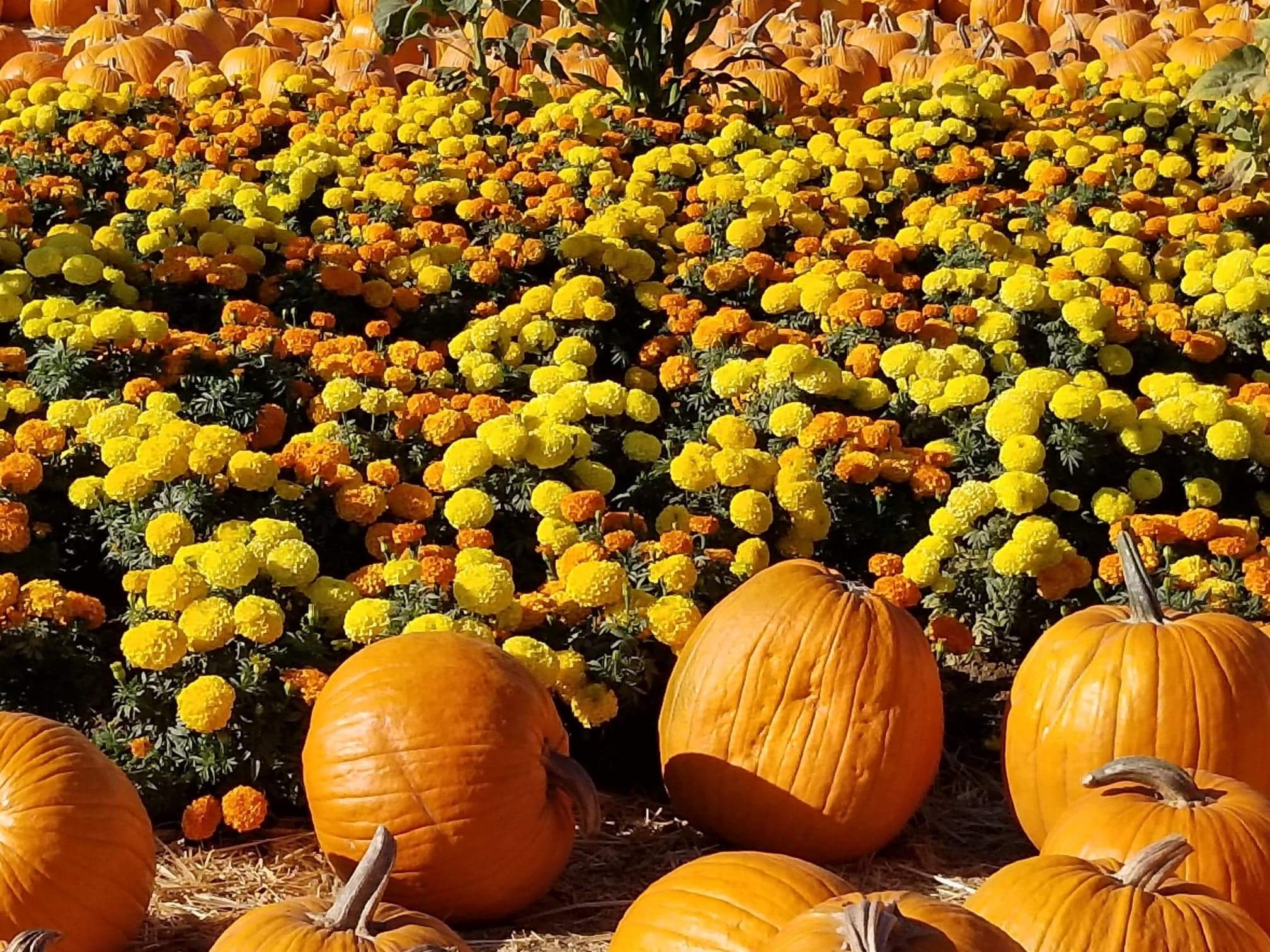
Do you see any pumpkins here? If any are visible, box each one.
[0,0,1270,123]
[0,714,155,952]
[207,531,1270,952]
